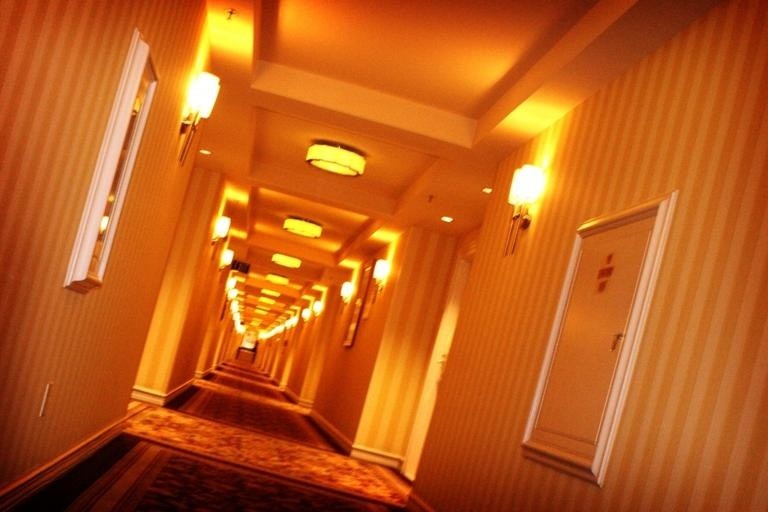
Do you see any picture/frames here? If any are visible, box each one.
[63,27,160,294]
[520,187,681,488]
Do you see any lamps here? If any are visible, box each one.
[176,72,546,345]
